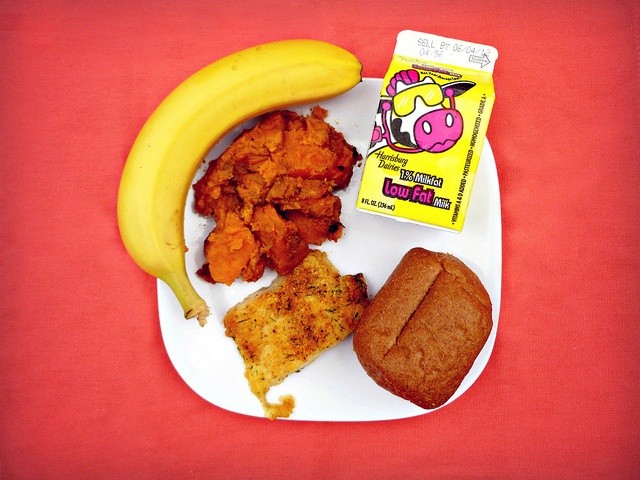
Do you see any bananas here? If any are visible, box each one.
[116,38,363,318]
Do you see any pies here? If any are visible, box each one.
[224,254,367,415]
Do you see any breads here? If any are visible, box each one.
[356,249,492,403]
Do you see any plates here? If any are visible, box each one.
[156,77,503,423]
